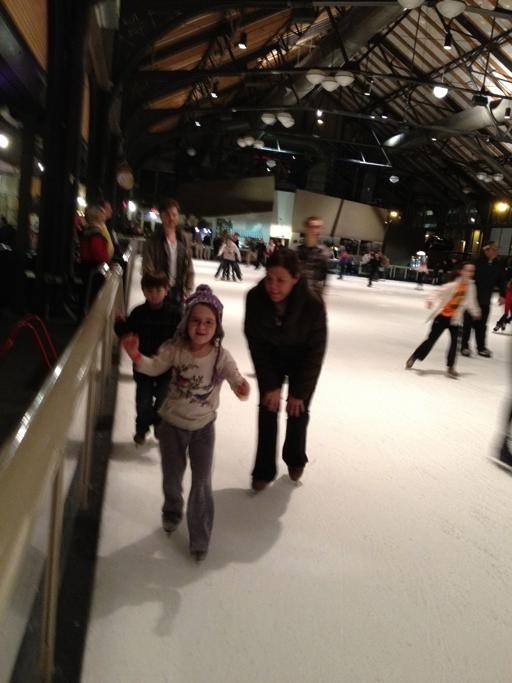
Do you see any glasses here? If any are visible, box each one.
[190,319,214,327]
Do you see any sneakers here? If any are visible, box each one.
[478,350,490,355]
[406,355,417,369]
[189,542,209,562]
[448,367,458,377]
[288,466,304,481]
[502,322,506,331]
[162,511,183,533]
[460,347,472,355]
[493,326,500,331]
[133,427,150,443]
[252,479,269,491]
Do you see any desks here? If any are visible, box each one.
[377,262,441,287]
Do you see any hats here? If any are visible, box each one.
[174,284,226,388]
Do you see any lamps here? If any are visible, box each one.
[112,129,136,194]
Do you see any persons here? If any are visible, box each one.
[70,197,283,449]
[122,284,251,561]
[243,248,327,492]
[404,241,512,472]
[291,216,391,294]
